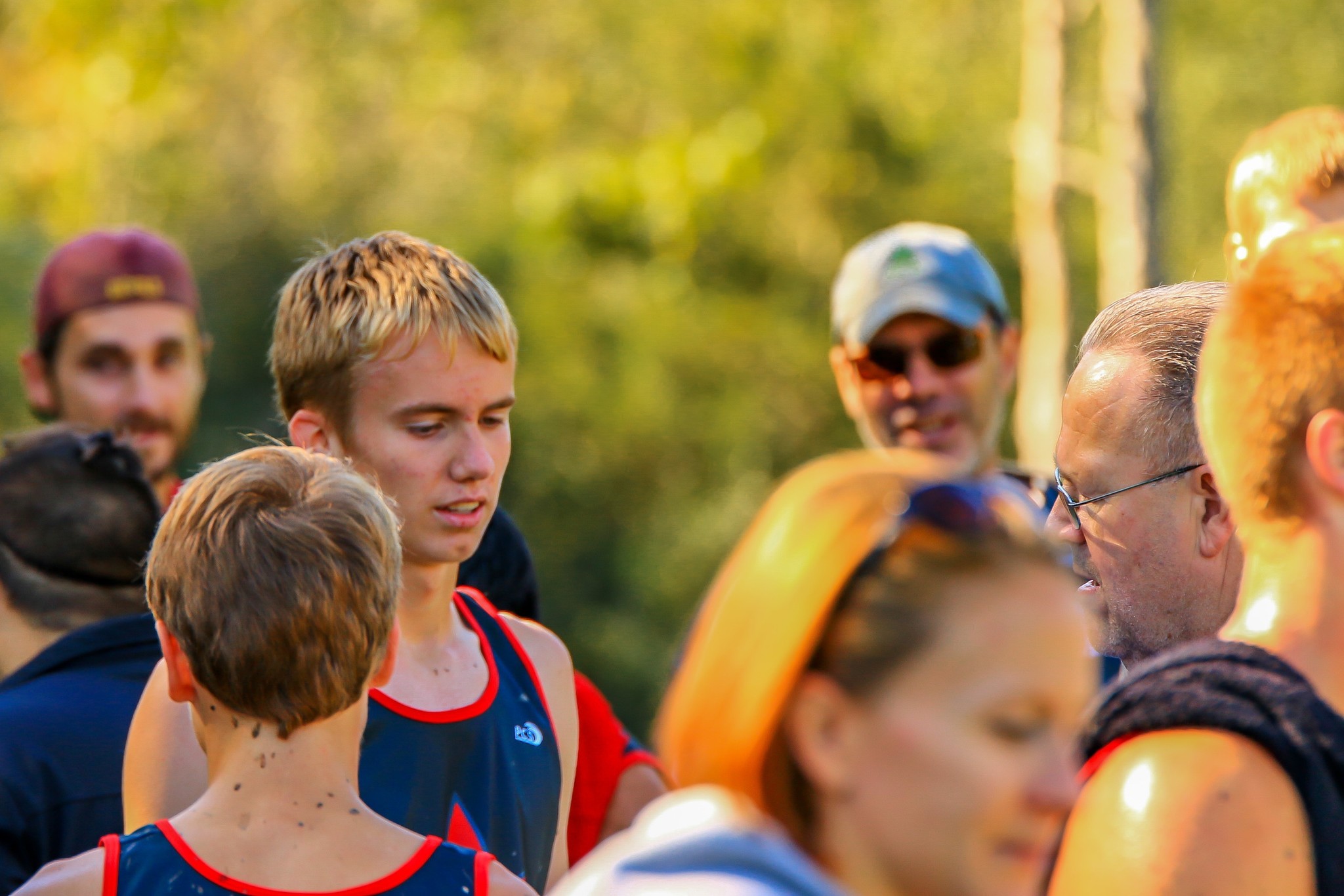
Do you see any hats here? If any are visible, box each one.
[833,220,1007,361]
[35,227,197,343]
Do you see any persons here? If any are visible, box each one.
[1,106,1344,896]
[536,446,1096,896]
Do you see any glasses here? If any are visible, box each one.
[1056,458,1204,530]
[809,475,1089,667]
[865,328,977,374]
